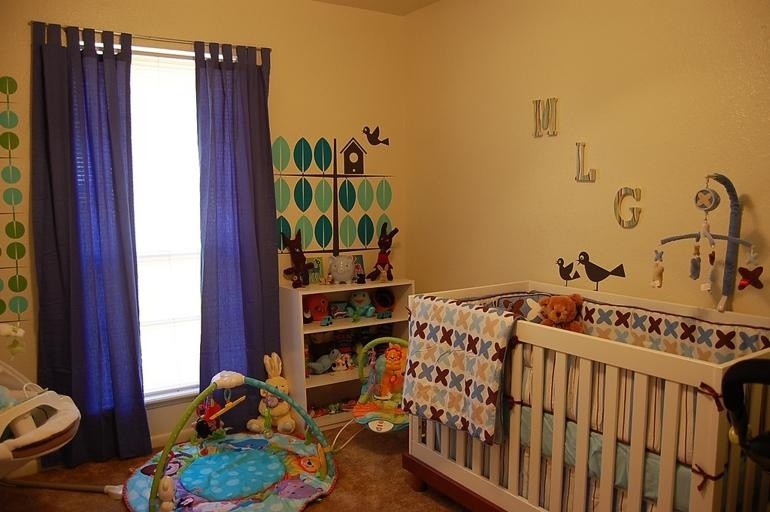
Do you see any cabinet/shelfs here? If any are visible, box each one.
[278,278,416,433]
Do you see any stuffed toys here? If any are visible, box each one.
[280,221,418,454]
[539,293,587,334]
[246,352,296,434]
[157,473,177,511]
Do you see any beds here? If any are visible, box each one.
[399,279,768,512]
